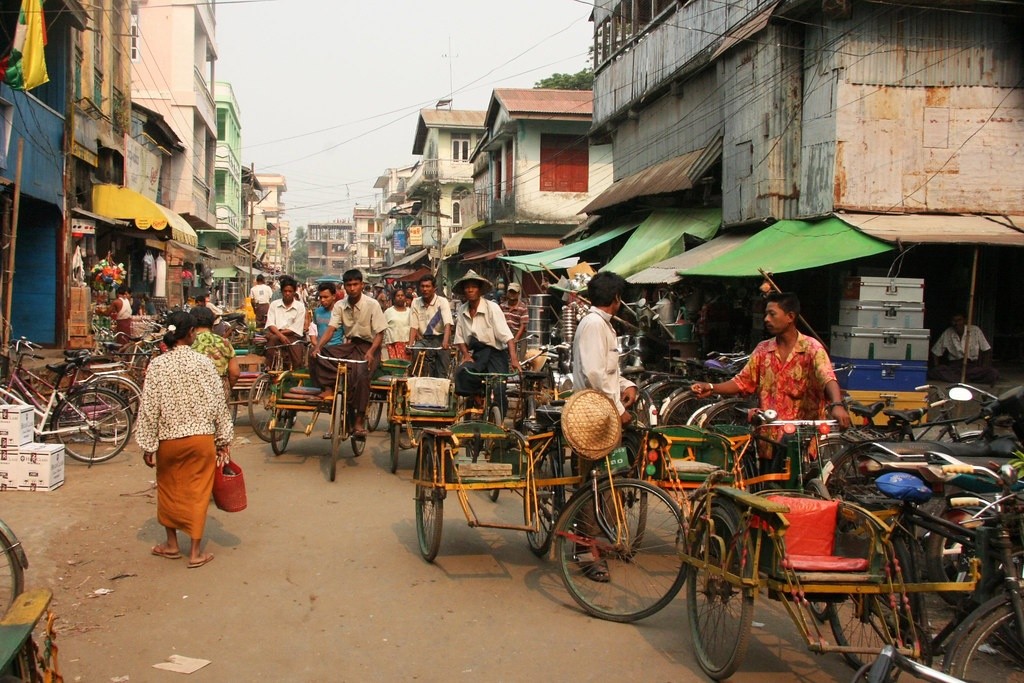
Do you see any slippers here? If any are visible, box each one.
[152,544,181,558]
[187,553,214,567]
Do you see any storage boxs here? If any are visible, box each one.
[67,285,95,348]
[0,404,65,493]
[829,276,931,427]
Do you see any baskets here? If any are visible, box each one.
[0,353,10,378]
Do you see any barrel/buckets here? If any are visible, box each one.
[528,294,551,345]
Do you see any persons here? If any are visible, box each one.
[569,272,638,581]
[306,269,388,438]
[133,311,234,569]
[404,275,454,378]
[250,274,420,335]
[313,282,351,346]
[383,288,422,361]
[195,295,224,338]
[925,312,1000,386]
[263,280,306,372]
[189,306,239,410]
[498,282,529,362]
[452,269,522,425]
[690,293,853,579]
[98,288,141,353]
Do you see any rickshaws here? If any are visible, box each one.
[227,333,1024,683]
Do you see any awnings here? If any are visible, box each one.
[837,212,1024,246]
[235,265,269,277]
[378,250,431,284]
[600,208,894,283]
[439,215,649,274]
[575,130,721,218]
[91,182,198,246]
[169,241,221,261]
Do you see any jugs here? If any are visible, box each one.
[626,297,692,343]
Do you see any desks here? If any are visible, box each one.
[669,340,699,360]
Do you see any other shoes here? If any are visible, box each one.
[354,428,367,437]
[578,552,610,582]
[594,542,618,559]
[312,391,334,400]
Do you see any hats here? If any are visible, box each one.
[524,349,549,371]
[508,282,520,292]
[562,388,621,461]
[451,270,493,295]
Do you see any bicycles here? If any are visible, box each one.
[0,315,166,468]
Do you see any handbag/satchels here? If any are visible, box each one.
[212,450,247,512]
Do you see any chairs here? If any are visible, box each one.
[753,492,892,585]
[273,373,329,412]
[434,421,523,484]
[400,376,455,417]
[654,423,733,482]
[371,358,409,389]
[236,354,266,390]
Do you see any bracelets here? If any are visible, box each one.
[708,383,714,396]
